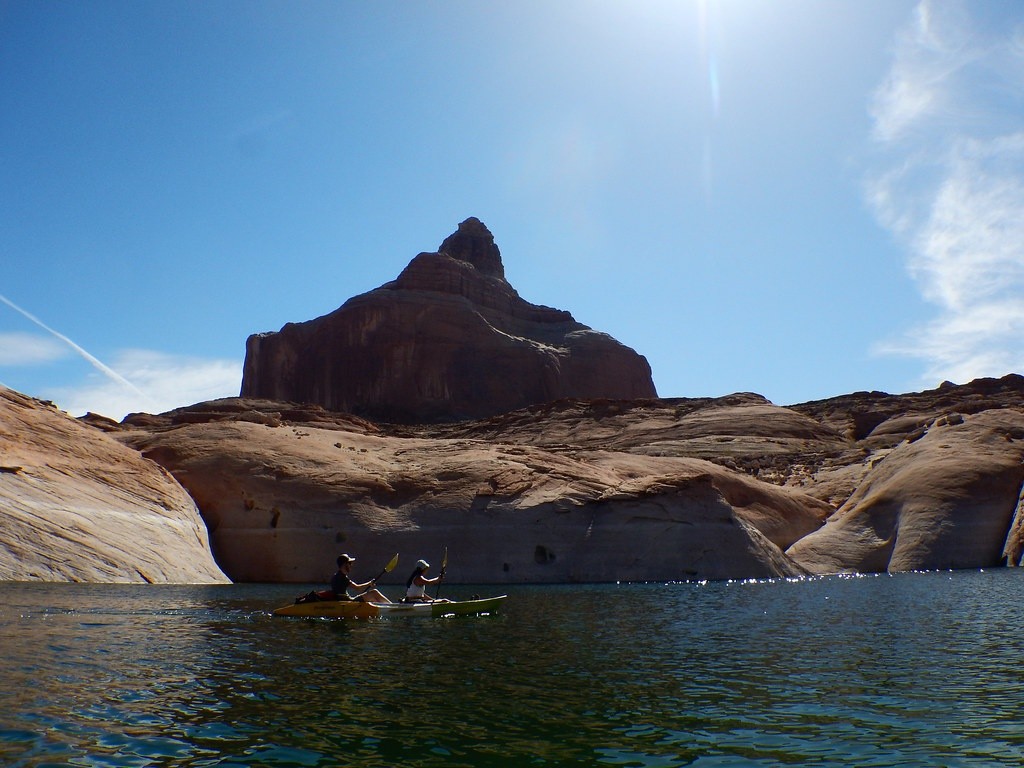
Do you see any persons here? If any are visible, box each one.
[330,554,405,603]
[405,560,480,603]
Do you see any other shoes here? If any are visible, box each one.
[475,594,480,600]
[470,595,475,600]
[398,598,402,603]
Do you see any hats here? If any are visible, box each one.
[337,554,356,565]
[416,560,430,570]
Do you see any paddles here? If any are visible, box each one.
[436,546,448,600]
[362,553,398,593]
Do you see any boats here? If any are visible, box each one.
[271,594,509,621]
